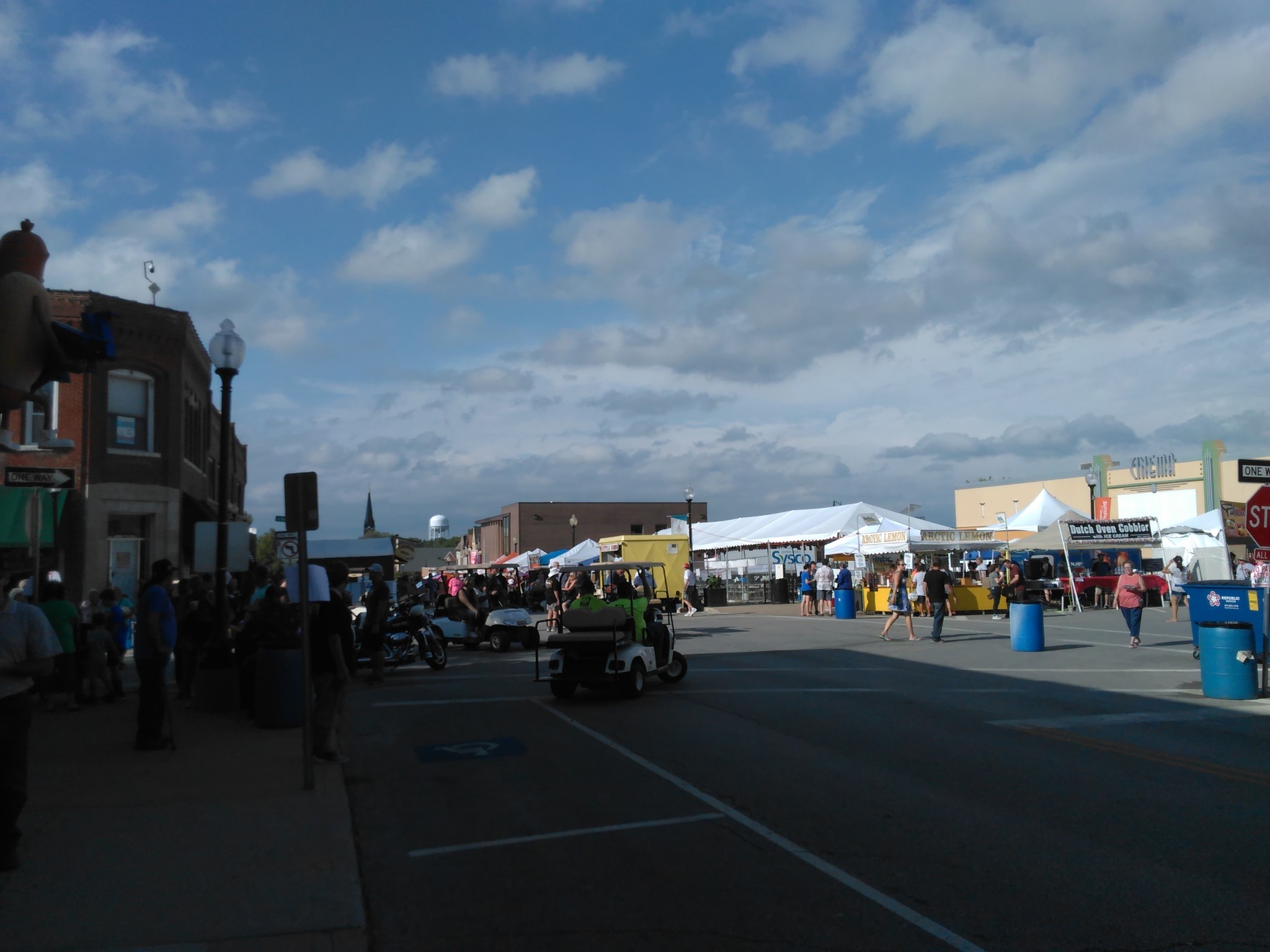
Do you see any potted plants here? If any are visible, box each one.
[702,573,731,607]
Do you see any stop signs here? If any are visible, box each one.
[1244,484,1270,548]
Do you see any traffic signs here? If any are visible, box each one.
[1237,458,1270,485]
[4,467,75,490]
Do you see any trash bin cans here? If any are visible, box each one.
[1198,620,1259,700]
[1009,599,1045,652]
[834,587,856,619]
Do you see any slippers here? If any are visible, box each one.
[1129,642,1143,648]
[1166,619,1178,623]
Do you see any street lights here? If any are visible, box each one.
[206,318,245,664]
[1086,465,1099,520]
[512,537,518,554]
[568,513,578,548]
[684,482,695,575]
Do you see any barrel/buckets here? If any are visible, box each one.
[1008,600,1045,652]
[834,587,856,619]
[1197,621,1259,700]
[771,578,789,604]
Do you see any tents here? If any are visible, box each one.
[997,508,1162,607]
[976,481,1090,532]
[652,501,957,603]
[494,538,602,575]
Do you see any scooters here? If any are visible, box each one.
[531,559,687,701]
[426,588,540,654]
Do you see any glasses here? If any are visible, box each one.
[899,564,906,566]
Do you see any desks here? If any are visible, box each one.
[1058,575,1169,609]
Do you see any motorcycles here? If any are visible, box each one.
[345,604,447,671]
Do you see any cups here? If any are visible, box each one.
[999,570,1004,577]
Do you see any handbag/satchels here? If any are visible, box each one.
[886,589,898,605]
[1185,567,1193,580]
[987,594,993,600]
[1138,598,1144,609]
[908,589,918,601]
[1244,576,1250,581]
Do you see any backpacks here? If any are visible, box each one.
[1002,585,1015,599]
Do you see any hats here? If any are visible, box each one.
[810,561,817,564]
[366,564,384,575]
[1097,553,1105,557]
[682,563,690,568]
[1235,556,1248,562]
[548,568,560,578]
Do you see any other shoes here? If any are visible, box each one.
[1094,604,1109,609]
[684,608,697,616]
[546,627,557,632]
[879,634,945,643]
[0,853,18,874]
[801,611,833,616]
[312,749,338,762]
[470,630,482,637]
[364,676,383,686]
[135,736,171,751]
[919,615,953,617]
[992,611,1009,620]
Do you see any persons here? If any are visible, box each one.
[0,561,853,689]
[1235,555,1255,580]
[132,558,183,750]
[878,558,922,641]
[1162,555,1191,622]
[1112,561,1147,648]
[309,561,349,763]
[1092,553,1111,609]
[923,557,957,643]
[883,552,1075,619]
[1230,550,1238,578]
[614,552,622,562]
[0,557,63,874]
